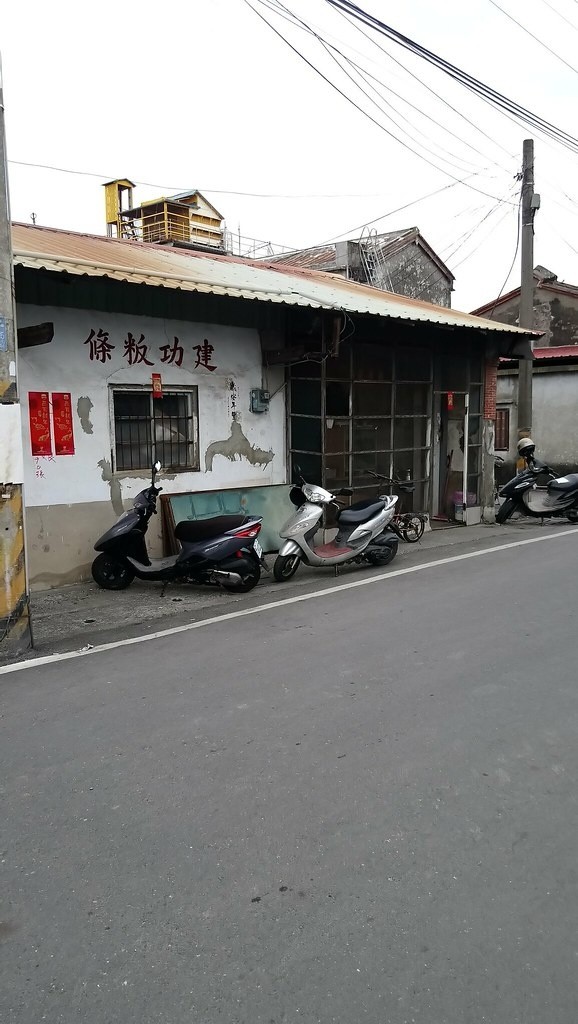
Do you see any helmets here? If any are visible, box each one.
[517,438,536,456]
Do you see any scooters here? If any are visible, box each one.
[495,452,578,524]
[273,464,400,582]
[91,459,270,598]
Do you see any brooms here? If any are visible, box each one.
[430,449,454,521]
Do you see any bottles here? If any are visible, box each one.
[405,469,410,481]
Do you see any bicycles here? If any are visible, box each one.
[367,469,427,543]
